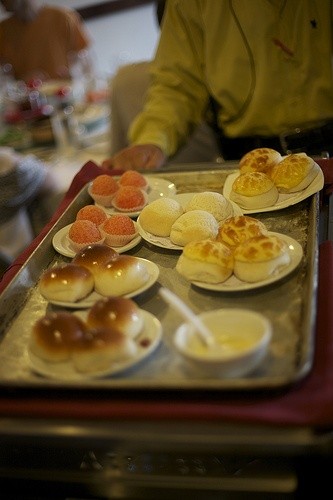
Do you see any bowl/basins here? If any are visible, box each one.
[174,309,270,378]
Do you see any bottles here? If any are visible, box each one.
[52,88,80,157]
[24,79,57,157]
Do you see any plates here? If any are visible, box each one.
[191,231,302,291]
[52,217,142,256]
[95,177,176,217]
[223,173,324,214]
[49,256,159,307]
[137,193,243,250]
[24,308,163,380]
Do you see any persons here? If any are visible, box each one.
[102,0,333,172]
[0,0,96,83]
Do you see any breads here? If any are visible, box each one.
[74,329,138,371]
[140,198,184,237]
[230,172,279,209]
[93,255,150,297]
[71,244,118,274]
[30,312,91,362]
[170,210,219,246]
[272,153,320,193]
[86,296,145,339]
[185,192,234,225]
[176,239,234,283]
[39,263,94,302]
[234,234,291,282]
[239,148,282,175]
[216,216,267,250]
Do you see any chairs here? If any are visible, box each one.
[109,60,221,167]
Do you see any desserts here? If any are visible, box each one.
[88,170,149,211]
[67,205,139,249]
